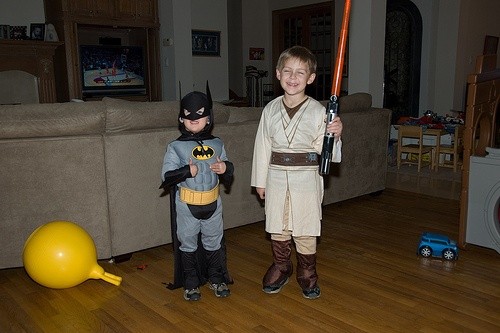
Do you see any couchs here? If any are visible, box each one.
[0,93,392,270]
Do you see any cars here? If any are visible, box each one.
[417,234,459,261]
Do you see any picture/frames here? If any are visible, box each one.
[190,28,222,57]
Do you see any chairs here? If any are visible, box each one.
[0,70,39,105]
[396,125,432,171]
[433,126,465,173]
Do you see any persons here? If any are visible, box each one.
[250,46,342,299]
[32,25,42,40]
[159,91,234,302]
[192,36,217,52]
[82,48,144,78]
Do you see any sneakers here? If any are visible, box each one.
[209,282,231,298]
[184,286,202,301]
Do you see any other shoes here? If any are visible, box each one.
[303,285,321,298]
[262,277,289,294]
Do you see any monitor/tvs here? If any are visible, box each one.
[79,44,148,94]
